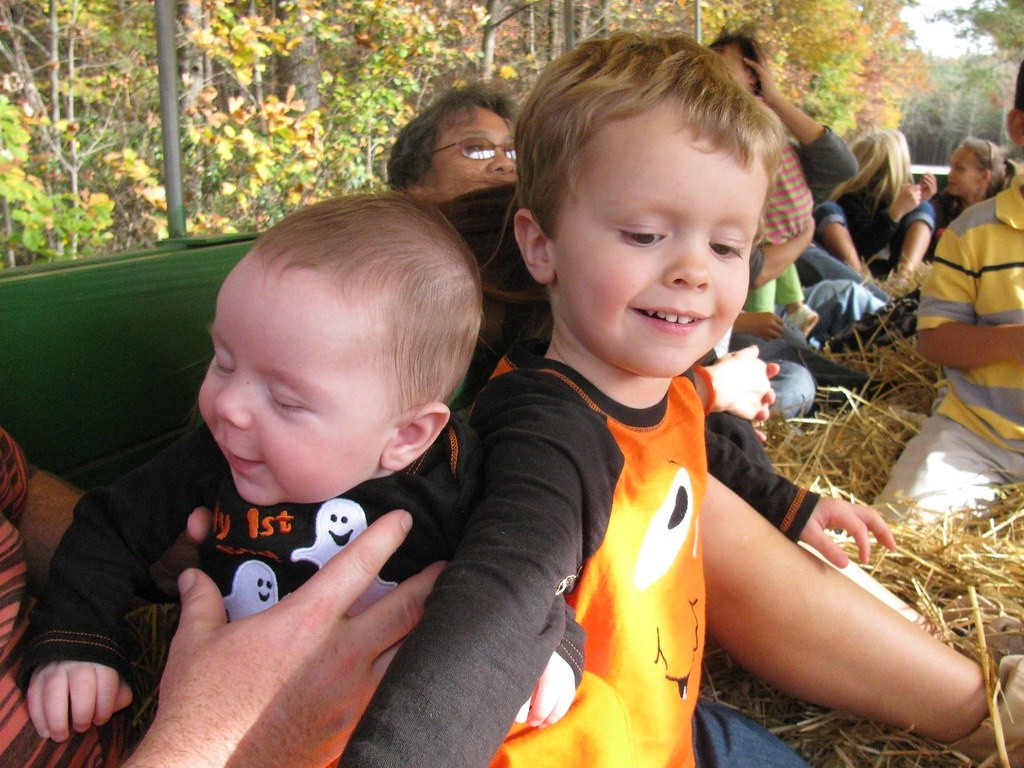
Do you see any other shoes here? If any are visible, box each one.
[783,305,820,338]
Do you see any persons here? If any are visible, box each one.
[10,191,587,742]
[0,428,448,768]
[340,30,897,768]
[708,31,913,425]
[438,184,1024,768]
[389,82,779,469]
[818,125,937,292]
[920,137,1018,262]
[875,62,1024,524]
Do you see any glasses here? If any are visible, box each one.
[432,136,516,161]
